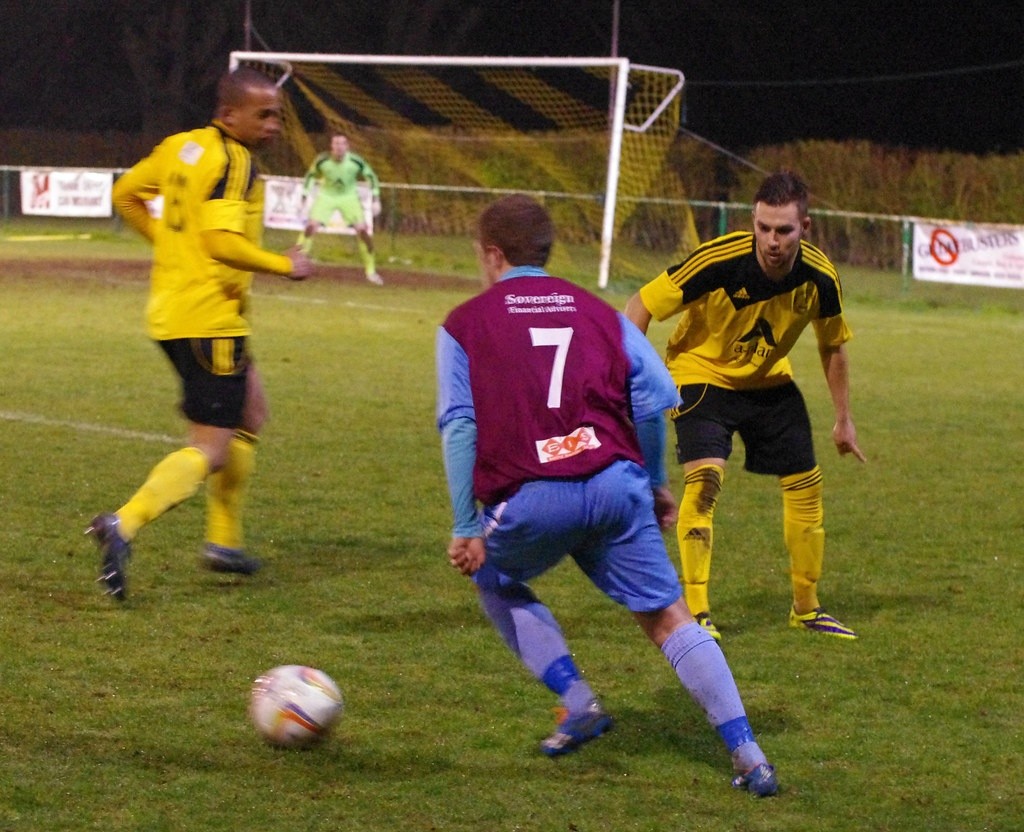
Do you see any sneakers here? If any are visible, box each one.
[542,704,612,756]
[789,604,858,639]
[205,545,258,573]
[697,614,721,640]
[86,512,130,597]
[733,762,780,796]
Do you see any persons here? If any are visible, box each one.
[296,133,385,285]
[436,193,781,800]
[84,68,319,605]
[623,170,867,641]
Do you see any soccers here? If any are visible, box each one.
[247,663,343,753]
